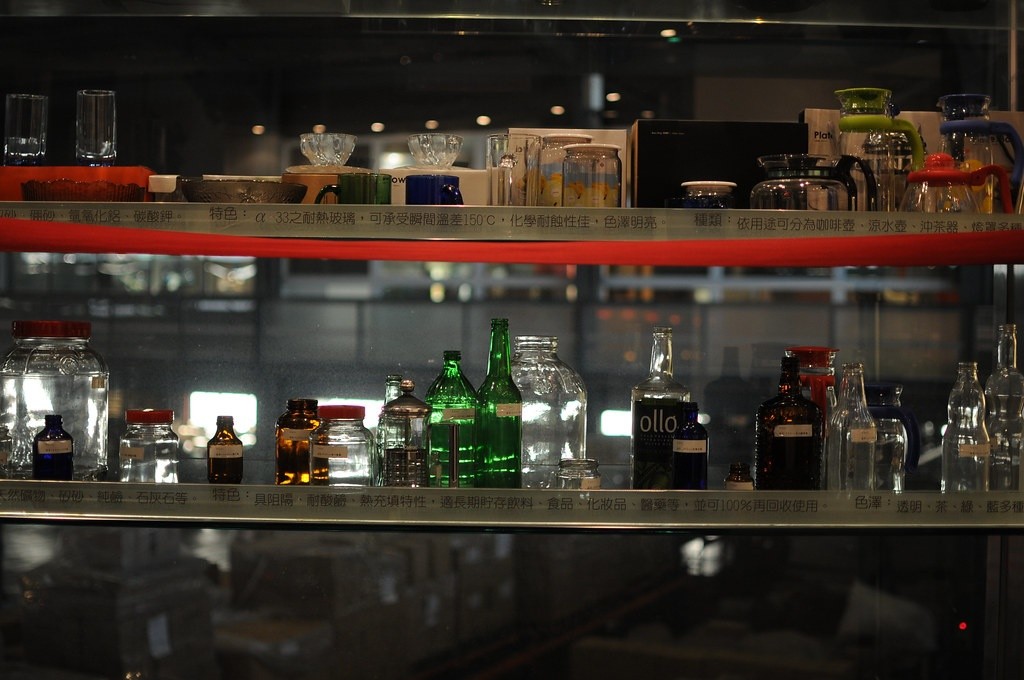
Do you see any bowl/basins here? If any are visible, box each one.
[21,177,145,202]
[299,132,358,166]
[407,133,464,167]
[180,176,308,204]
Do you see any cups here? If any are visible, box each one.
[4,94,48,167]
[485,133,623,208]
[313,173,392,205]
[405,174,464,205]
[75,90,117,167]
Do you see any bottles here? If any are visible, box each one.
[723,462,755,491]
[755,354,920,491]
[206,416,244,485]
[0,318,109,483]
[118,409,180,484]
[275,398,376,487]
[673,402,708,490]
[983,323,1024,492]
[375,350,477,488]
[940,361,990,493]
[474,318,523,488]
[510,334,588,489]
[557,458,601,491]
[631,325,691,490]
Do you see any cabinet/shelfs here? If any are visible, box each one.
[1,198,1024,537]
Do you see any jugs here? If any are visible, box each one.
[833,87,925,213]
[898,152,1015,214]
[935,94,1024,215]
[680,181,737,209]
[749,153,879,212]
